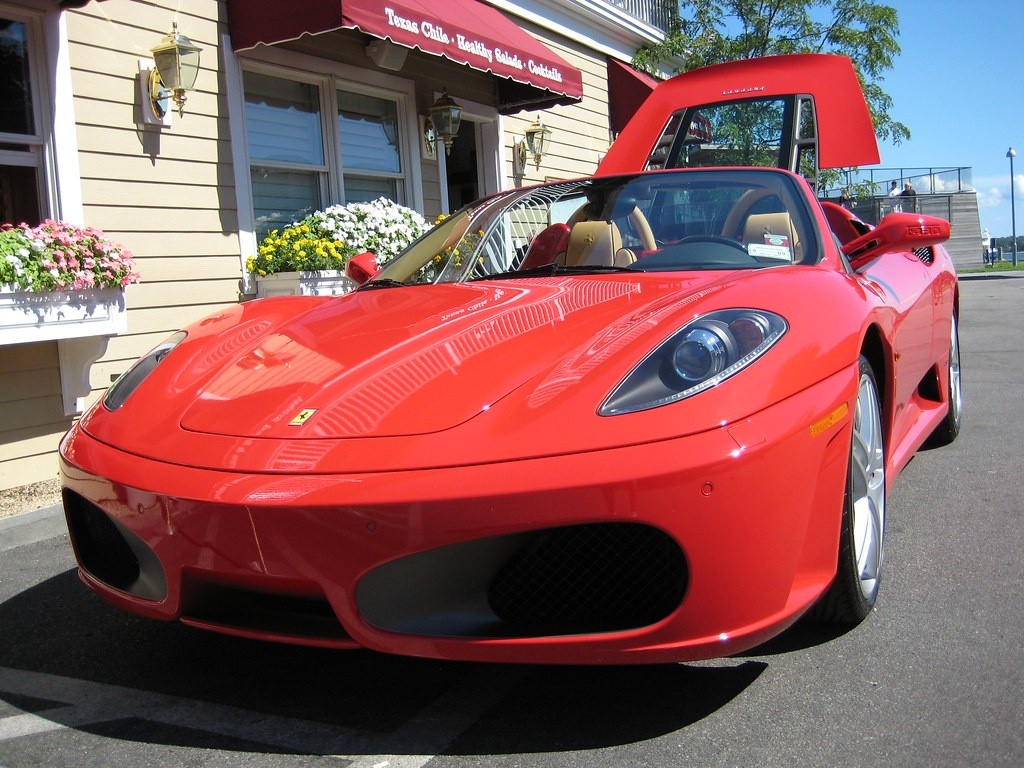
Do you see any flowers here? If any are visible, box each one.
[0,219,141,293]
[245,195,485,281]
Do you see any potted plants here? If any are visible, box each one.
[254,269,360,296]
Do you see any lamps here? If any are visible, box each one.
[417,87,464,159]
[514,114,552,175]
[139,21,204,124]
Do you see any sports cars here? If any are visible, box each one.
[55,54,963,668]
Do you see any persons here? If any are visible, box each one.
[888,180,903,215]
[900,182,919,213]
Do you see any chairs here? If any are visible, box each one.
[554,200,657,269]
[721,187,804,266]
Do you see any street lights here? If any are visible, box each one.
[1006,147,1017,267]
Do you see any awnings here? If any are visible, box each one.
[605,54,713,146]
[225,0,583,115]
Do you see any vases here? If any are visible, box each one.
[0,284,126,347]
